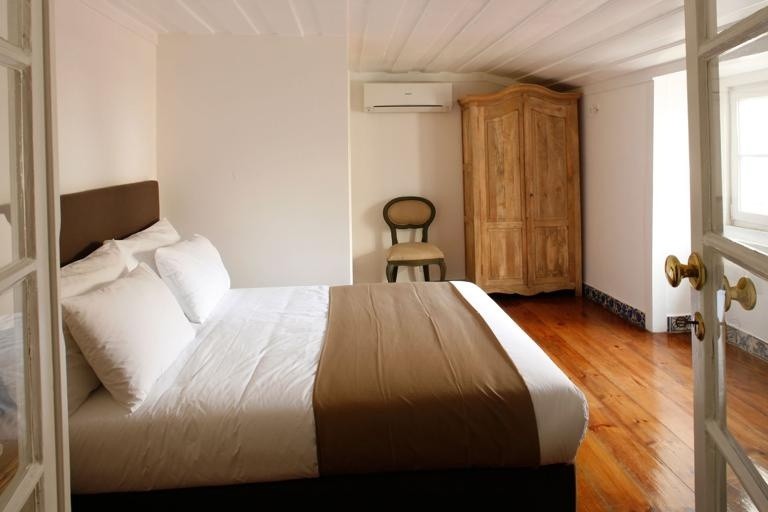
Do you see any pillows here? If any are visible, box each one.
[154,233,231,323]
[104,217,182,278]
[0,239,129,440]
[60,262,196,413]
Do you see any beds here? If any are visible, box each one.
[0,180,589,512]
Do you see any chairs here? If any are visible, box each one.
[383,196,447,282]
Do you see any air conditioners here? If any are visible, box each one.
[363,82,453,112]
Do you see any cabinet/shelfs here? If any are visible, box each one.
[457,83,582,297]
